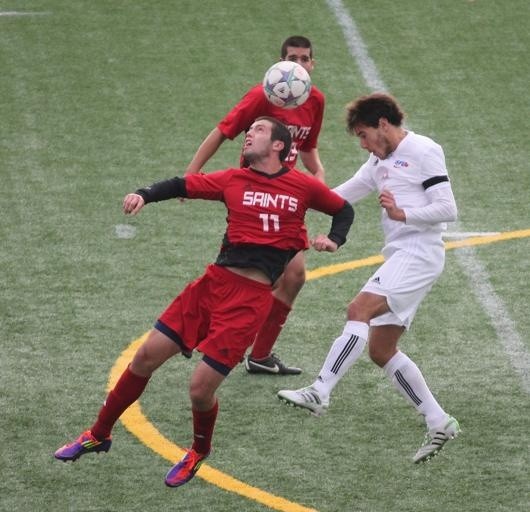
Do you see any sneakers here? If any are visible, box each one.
[165,446,212,488]
[55,431,113,462]
[245,352,303,376]
[413,414,463,465]
[277,386,329,418]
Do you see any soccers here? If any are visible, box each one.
[263,61,311,108]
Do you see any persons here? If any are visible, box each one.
[171,36,326,375]
[54,114,355,488]
[277,94,461,465]
